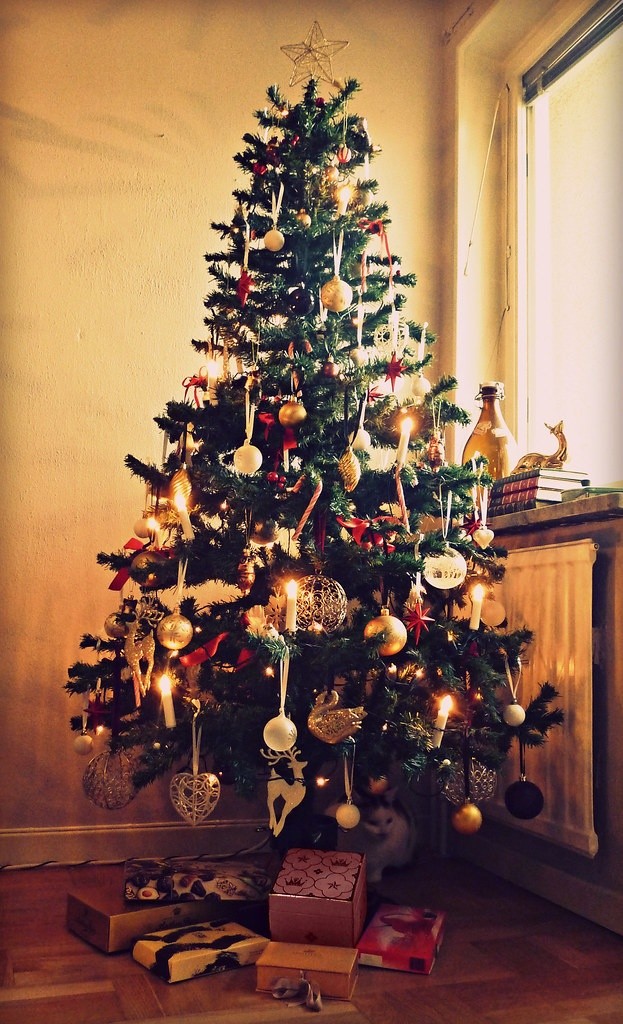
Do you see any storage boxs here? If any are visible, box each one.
[123,857,276,902]
[267,848,369,948]
[255,941,360,1001]
[357,904,449,974]
[131,918,268,983]
[65,886,224,953]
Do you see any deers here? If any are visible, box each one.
[510,420,568,476]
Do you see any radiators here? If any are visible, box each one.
[454,540,603,859]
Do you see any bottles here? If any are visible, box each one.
[462,382,519,480]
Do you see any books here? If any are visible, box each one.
[488,468,589,517]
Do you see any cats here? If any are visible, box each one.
[322,785,420,885]
